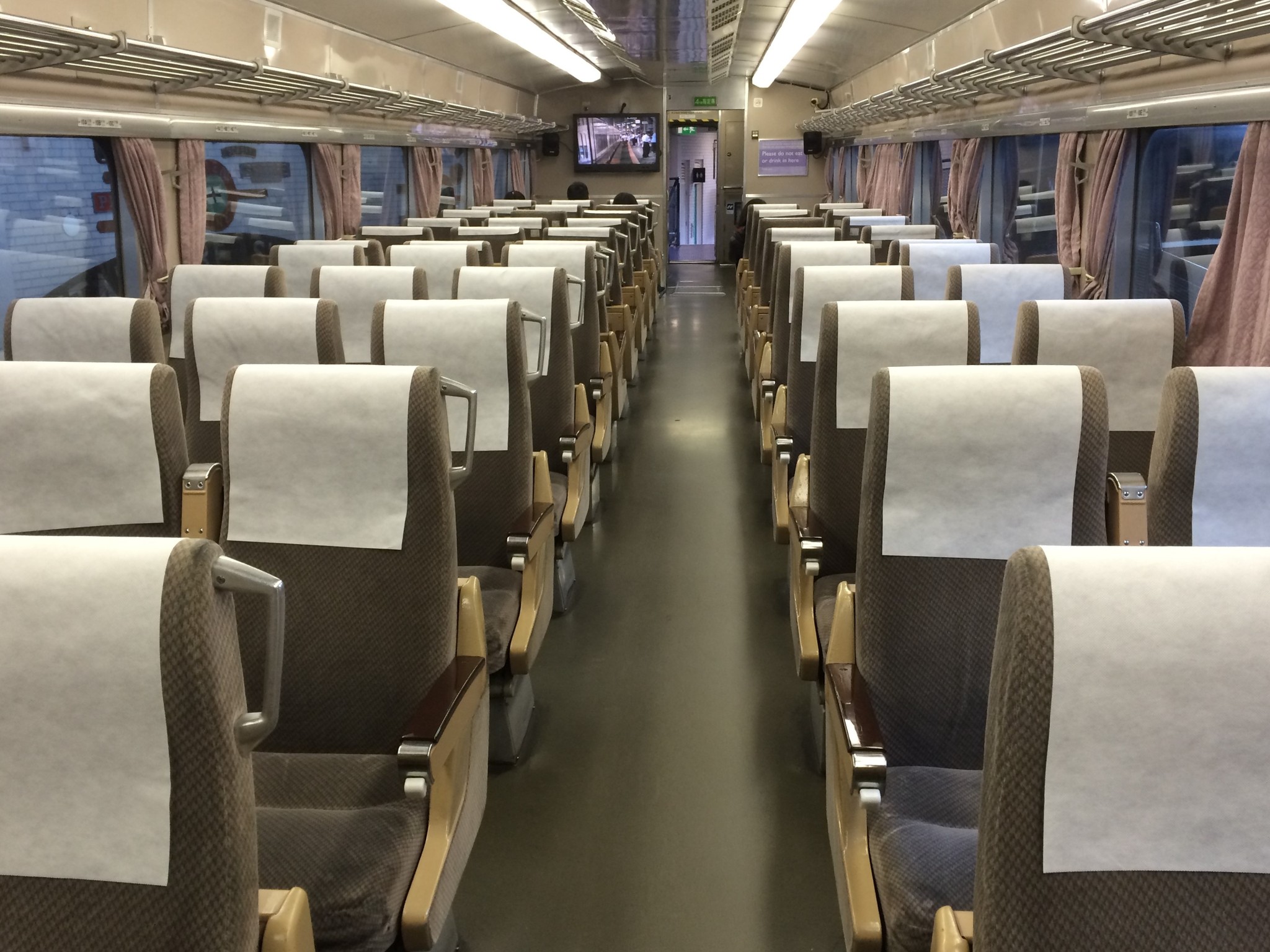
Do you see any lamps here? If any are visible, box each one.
[433,0,603,83]
[751,0,842,88]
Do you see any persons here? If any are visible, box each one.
[503,190,525,200]
[567,181,590,200]
[641,131,651,158]
[613,192,666,300]
[621,131,645,148]
[650,132,656,153]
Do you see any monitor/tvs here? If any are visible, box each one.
[573,113,661,174]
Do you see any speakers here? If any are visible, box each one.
[804,131,822,155]
[542,133,560,156]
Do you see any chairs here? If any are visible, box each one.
[0,190,457,361]
[0,198,663,952]
[941,165,1236,337]
[734,202,1270,952]
